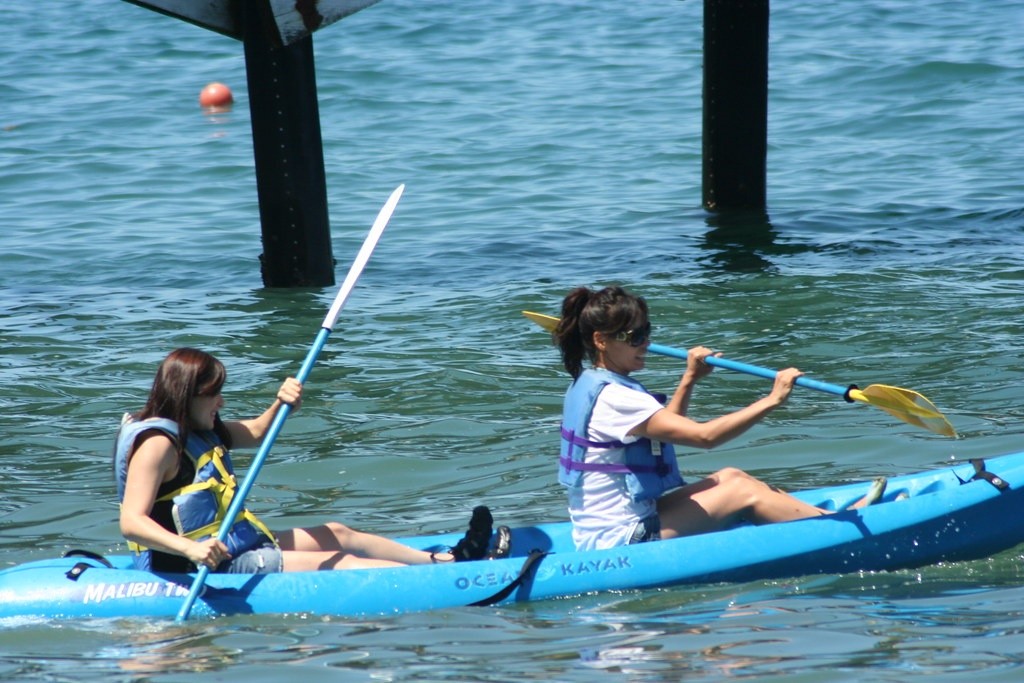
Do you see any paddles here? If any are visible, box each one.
[178,181,414,621]
[521,306,957,435]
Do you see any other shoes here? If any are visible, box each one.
[895,493,908,502]
[481,526,511,559]
[846,478,887,511]
[450,506,493,561]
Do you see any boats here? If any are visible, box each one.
[0,452,1023,620]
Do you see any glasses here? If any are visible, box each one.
[606,322,651,347]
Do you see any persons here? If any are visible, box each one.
[115,348,511,573]
[558,284,908,552]
